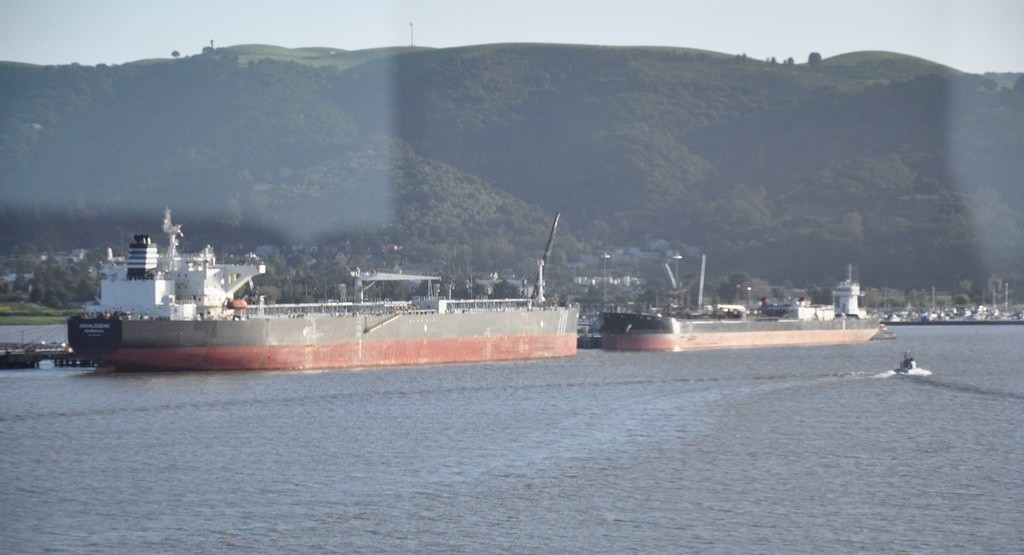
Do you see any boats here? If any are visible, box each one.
[894,361,931,377]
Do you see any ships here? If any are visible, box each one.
[67,205,583,375]
[597,264,880,352]
